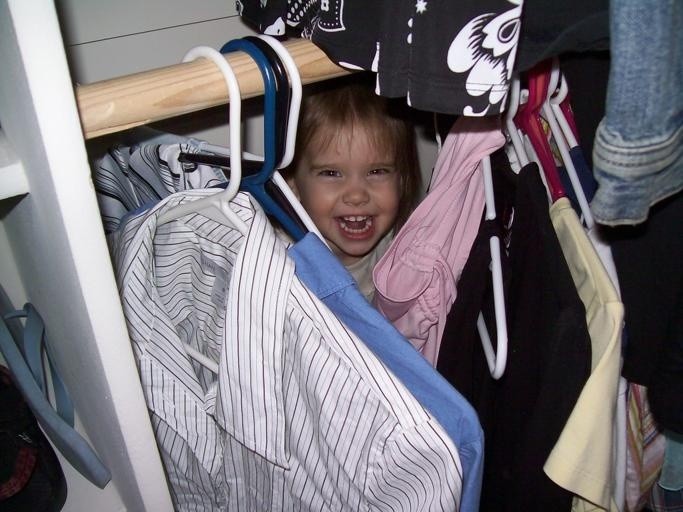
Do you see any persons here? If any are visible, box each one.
[277,71,421,313]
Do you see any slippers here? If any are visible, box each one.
[1,285,112,512]
